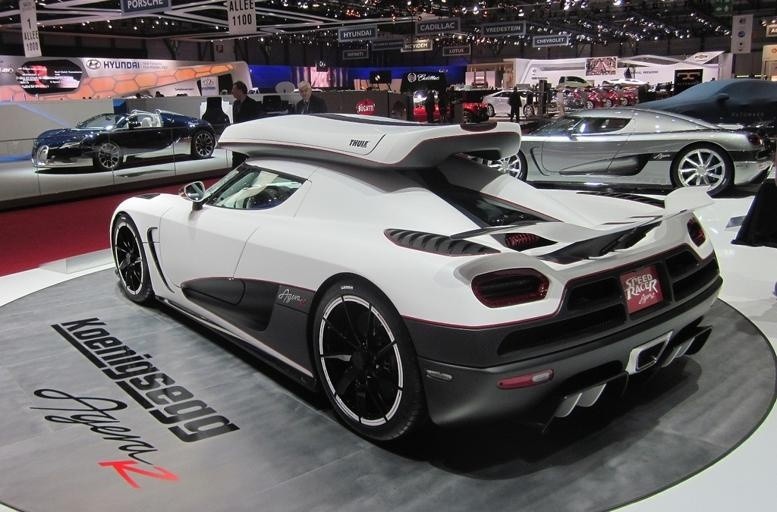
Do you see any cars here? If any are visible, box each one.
[485,80,777,187]
[412,83,538,123]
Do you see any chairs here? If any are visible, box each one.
[141,117,152,128]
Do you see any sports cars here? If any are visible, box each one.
[110,114,723,452]
[32,109,216,171]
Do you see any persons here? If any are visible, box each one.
[295,80,326,115]
[507,86,522,123]
[231,82,266,171]
[425,91,436,124]
[437,86,449,125]
